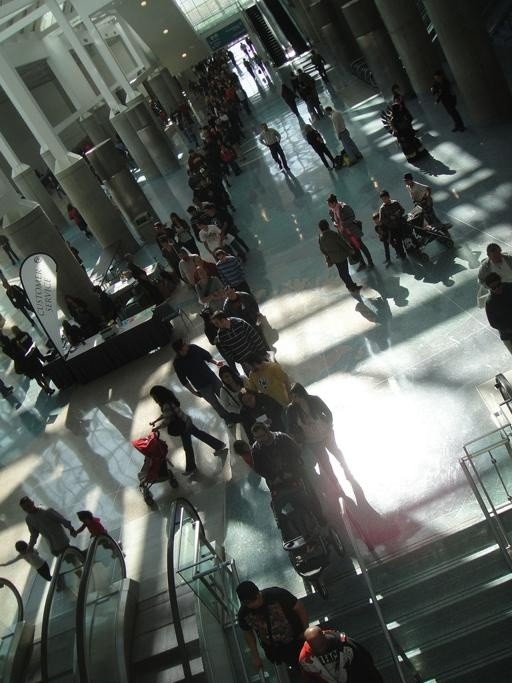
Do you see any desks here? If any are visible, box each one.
[63,300,176,386]
[40,332,74,391]
[103,260,166,308]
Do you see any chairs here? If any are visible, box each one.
[151,300,193,334]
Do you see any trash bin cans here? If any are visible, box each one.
[133,211,157,246]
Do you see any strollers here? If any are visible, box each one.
[132,421,180,508]
[267,465,346,585]
[401,205,457,263]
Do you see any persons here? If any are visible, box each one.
[281,48,363,170]
[389,82,430,164]
[238,580,385,683]
[431,70,466,133]
[148,249,353,529]
[259,123,290,172]
[478,243,511,352]
[0,103,199,408]
[186,49,250,261]
[0,498,126,592]
[318,173,451,291]
[240,37,267,82]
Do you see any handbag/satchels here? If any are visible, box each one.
[167,418,185,436]
